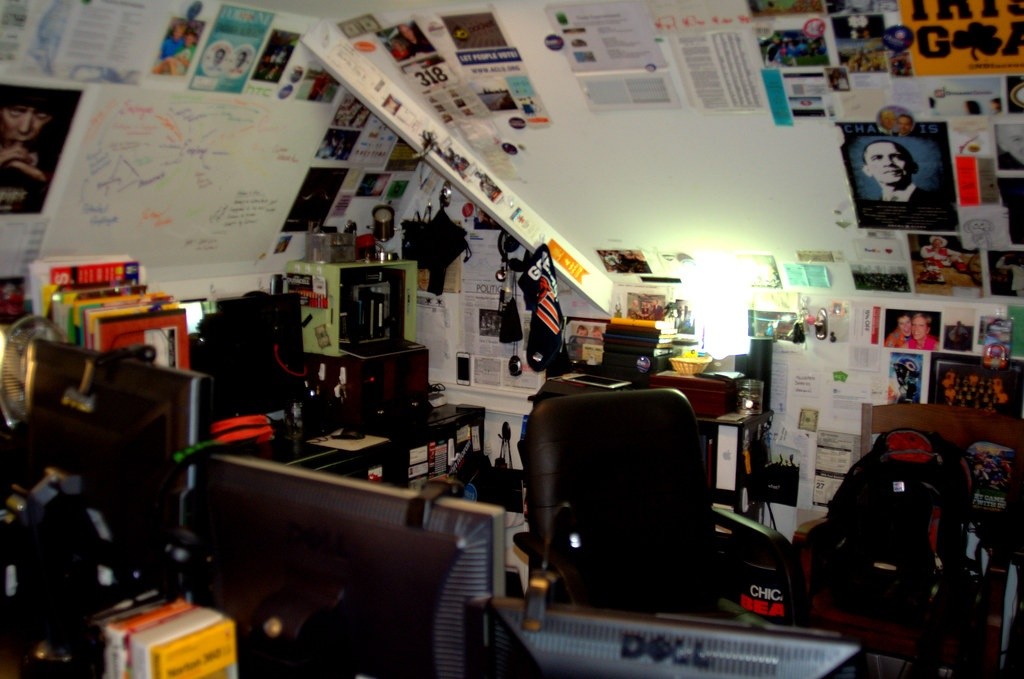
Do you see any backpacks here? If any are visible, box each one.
[809,429,978,642]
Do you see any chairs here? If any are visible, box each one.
[794,404,1024,679]
[513,389,811,629]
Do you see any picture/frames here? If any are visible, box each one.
[564,316,609,364]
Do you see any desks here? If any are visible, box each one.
[528,396,774,523]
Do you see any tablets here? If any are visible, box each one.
[562,374,632,389]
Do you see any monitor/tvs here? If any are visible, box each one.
[23,336,214,549]
[210,292,305,421]
[464,595,868,679]
[189,452,506,679]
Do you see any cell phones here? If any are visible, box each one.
[456,352,470,386]
